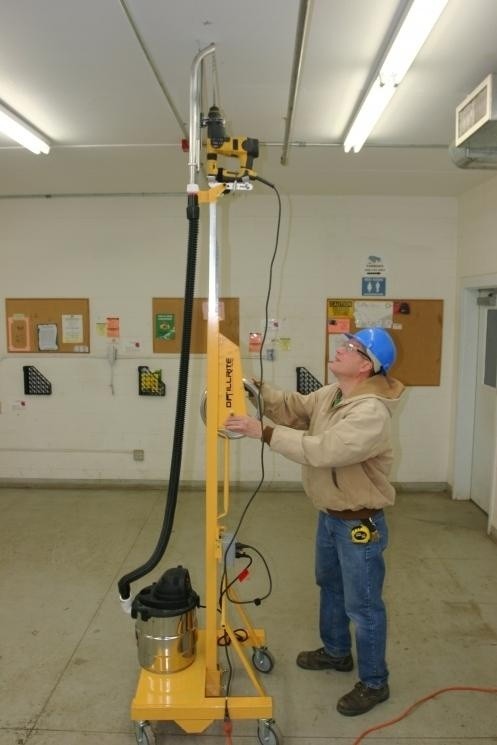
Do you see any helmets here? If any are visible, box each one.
[343,325,396,378]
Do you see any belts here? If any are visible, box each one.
[323,507,381,522]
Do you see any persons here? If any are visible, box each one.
[224,328,406,716]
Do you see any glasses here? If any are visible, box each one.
[341,342,371,361]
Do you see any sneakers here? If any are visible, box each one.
[295,646,354,672]
[337,679,390,717]
